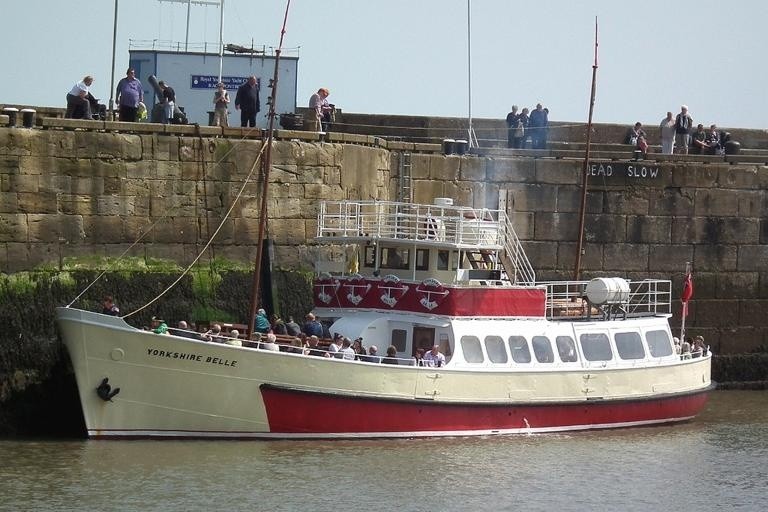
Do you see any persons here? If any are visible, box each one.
[675,105,693,154]
[115,68,144,132]
[308,88,330,143]
[507,103,549,148]
[322,88,332,143]
[621,122,648,157]
[710,123,721,154]
[144,304,446,368]
[159,80,175,122]
[693,125,712,155]
[656,112,675,155]
[672,335,707,360]
[64,76,94,120]
[235,75,260,126]
[102,294,121,315]
[212,83,232,127]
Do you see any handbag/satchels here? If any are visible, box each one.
[514,121,524,138]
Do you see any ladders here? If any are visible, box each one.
[400,151,413,238]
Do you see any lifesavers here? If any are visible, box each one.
[377,276,403,304]
[418,279,443,310]
[342,274,366,302]
[315,272,336,301]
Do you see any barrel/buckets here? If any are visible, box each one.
[441,138,455,154]
[456,137,469,155]
[586,277,630,304]
[424,215,462,238]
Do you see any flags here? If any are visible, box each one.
[679,270,696,320]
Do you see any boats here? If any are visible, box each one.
[56,200,718,439]
